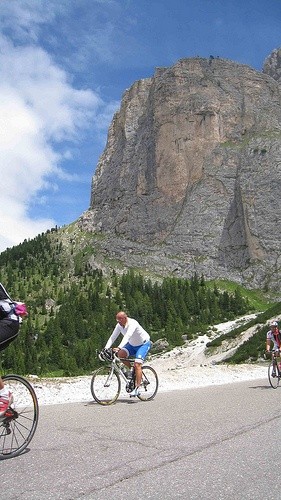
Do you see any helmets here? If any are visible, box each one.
[269,321,278,326]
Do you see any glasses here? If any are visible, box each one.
[116,317,126,322]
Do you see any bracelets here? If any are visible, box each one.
[118,347,121,350]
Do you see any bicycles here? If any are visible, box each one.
[0,374,39,460]
[91,348,159,406]
[263,349,281,389]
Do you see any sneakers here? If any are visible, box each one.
[127,366,134,379]
[0,391,13,417]
[129,387,141,395]
[278,372,281,380]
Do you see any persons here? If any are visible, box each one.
[101,312,151,395]
[265,322,281,377]
[0,283,23,421]
